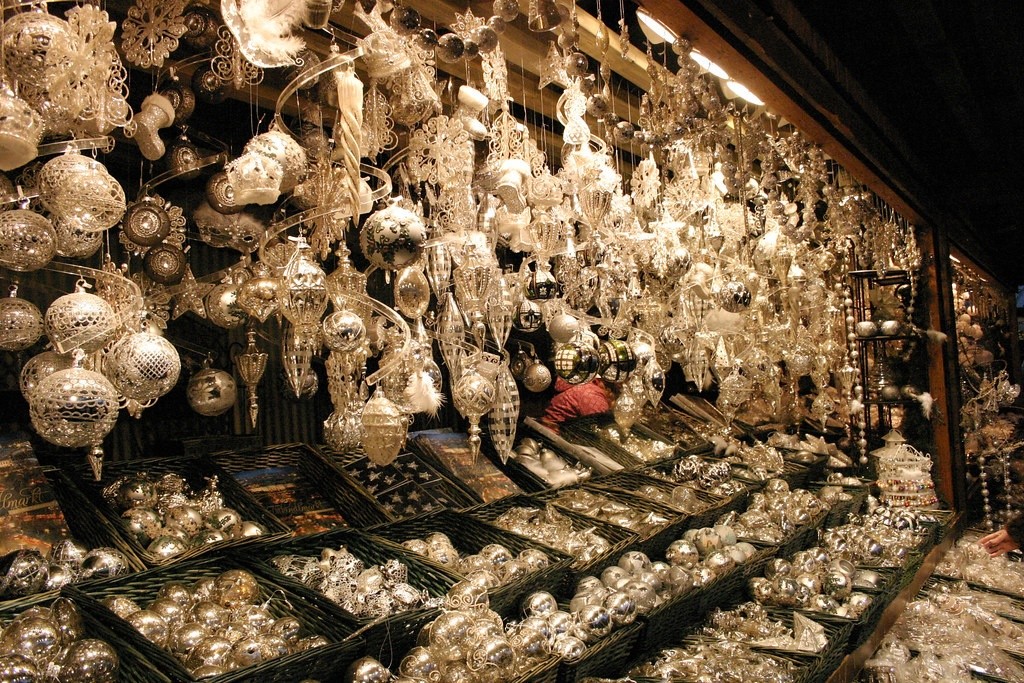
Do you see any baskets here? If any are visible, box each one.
[0,401,1024,683]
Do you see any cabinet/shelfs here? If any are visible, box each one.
[849,267,932,454]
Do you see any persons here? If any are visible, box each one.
[546,376,611,423]
[976,514,1024,559]
